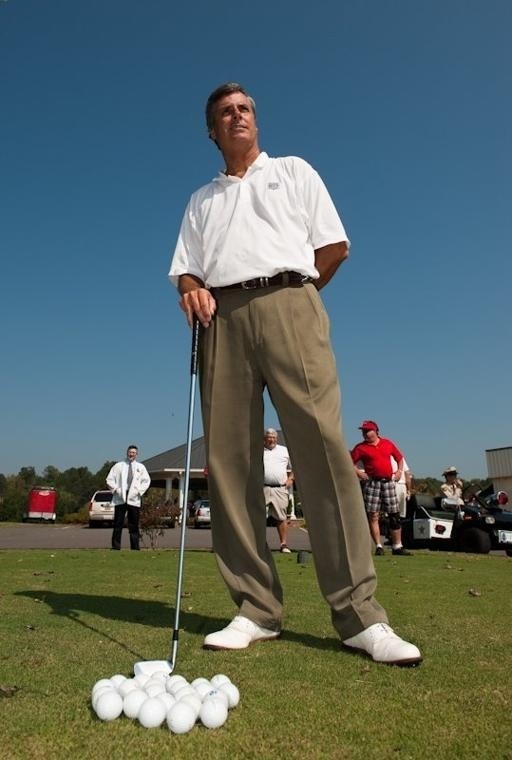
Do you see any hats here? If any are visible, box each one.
[442,466,458,476]
[358,420,379,431]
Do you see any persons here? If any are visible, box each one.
[106,446,151,550]
[351,421,415,555]
[260,428,296,554]
[440,466,479,514]
[385,454,413,546]
[169,84,424,667]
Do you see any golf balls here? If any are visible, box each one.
[90,673,240,734]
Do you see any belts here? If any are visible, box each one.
[217,270,308,292]
[263,483,285,487]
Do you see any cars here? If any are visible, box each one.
[190,498,211,528]
[160,514,179,529]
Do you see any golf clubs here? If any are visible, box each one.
[134,311,200,674]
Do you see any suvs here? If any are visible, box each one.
[87,490,127,525]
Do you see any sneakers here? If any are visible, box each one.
[280,544,291,553]
[376,547,384,555]
[391,547,412,556]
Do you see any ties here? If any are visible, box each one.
[128,462,133,489]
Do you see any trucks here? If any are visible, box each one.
[22,485,57,524]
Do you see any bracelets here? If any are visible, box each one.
[397,469,402,473]
[289,477,294,480]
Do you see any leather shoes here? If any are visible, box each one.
[342,623,424,665]
[204,615,281,651]
